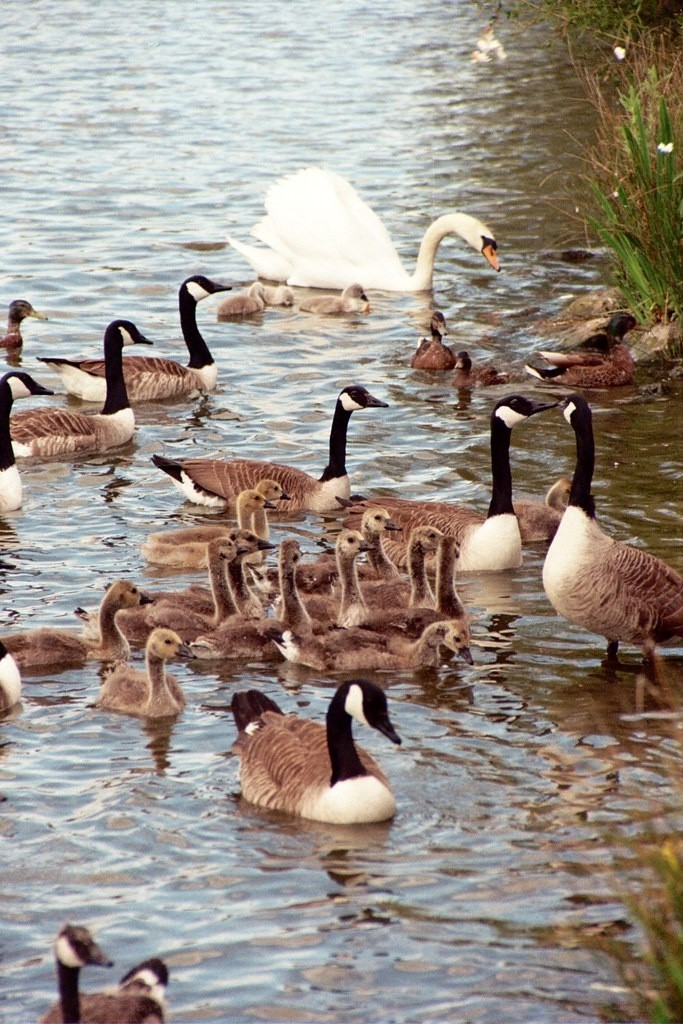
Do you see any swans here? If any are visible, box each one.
[224,167,502,291]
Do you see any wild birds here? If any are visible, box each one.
[409,310,456,371]
[243,284,294,307]
[449,352,519,389]
[524,311,648,386]
[507,479,572,543]
[37,275,233,402]
[0,580,154,667]
[8,319,153,458]
[218,281,265,315]
[331,396,559,572]
[0,300,48,349]
[0,639,23,712]
[94,628,198,717]
[153,386,391,513]
[299,283,369,314]
[38,925,169,1024]
[541,396,683,667]
[66,480,476,674]
[1,371,54,512]
[223,676,402,823]
